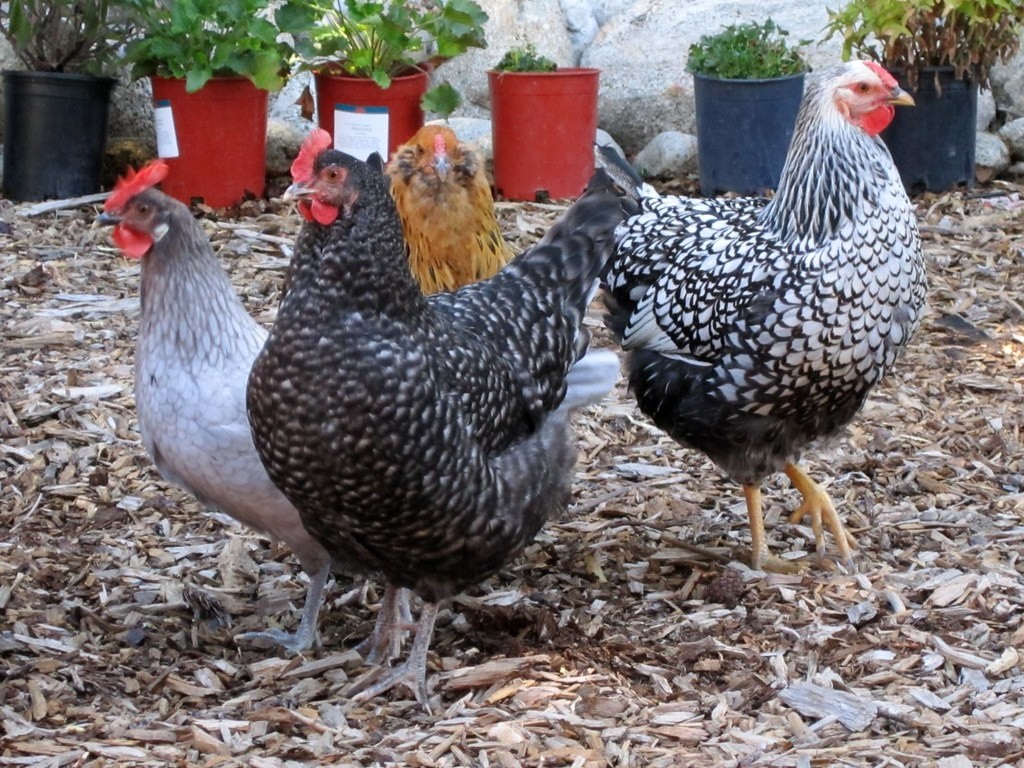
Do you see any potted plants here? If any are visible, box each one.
[683,16,811,199]
[817,0,1024,197]
[274,1,488,168]
[484,40,602,204]
[123,0,294,211]
[2,1,133,204]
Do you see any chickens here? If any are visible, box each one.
[592,57,929,582]
[95,159,379,658]
[379,123,513,300]
[248,128,624,719]
